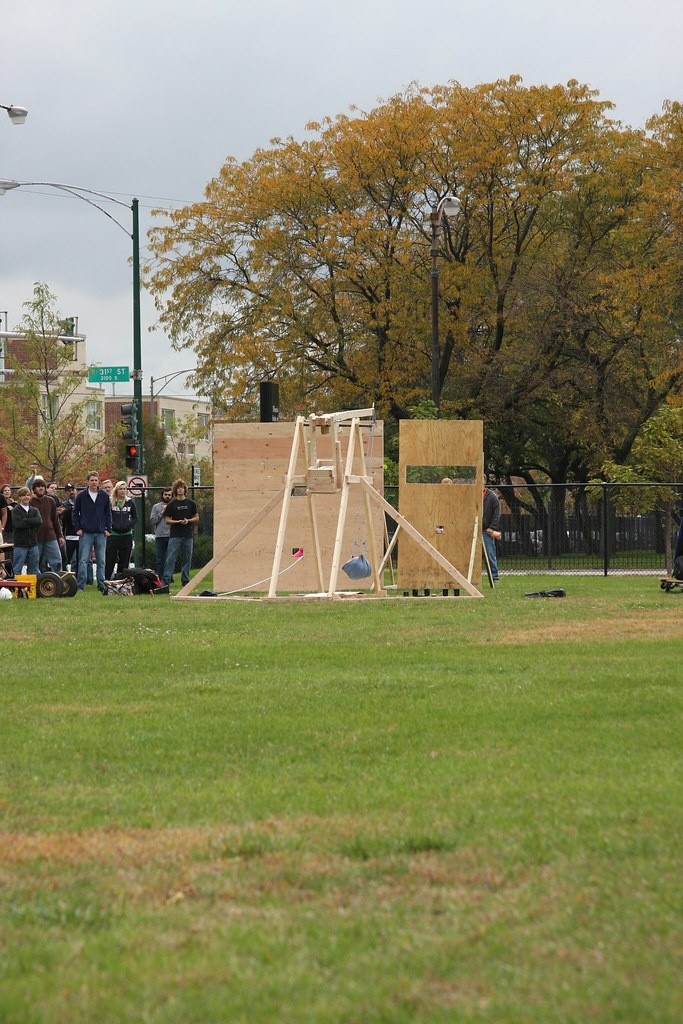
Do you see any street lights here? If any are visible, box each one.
[149,367,210,426]
[1,178,148,591]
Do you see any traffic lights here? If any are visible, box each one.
[121,404,134,440]
[57,317,75,360]
[124,444,140,468]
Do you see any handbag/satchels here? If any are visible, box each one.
[104,577,133,596]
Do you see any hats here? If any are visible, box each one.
[65,483,75,491]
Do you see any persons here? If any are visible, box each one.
[483,473,501,582]
[150,478,199,587]
[0,465,139,593]
